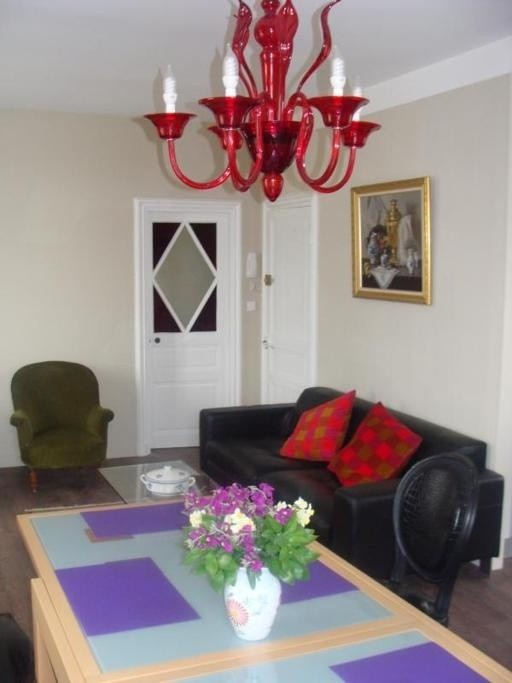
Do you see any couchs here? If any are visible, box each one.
[197,385,504,581]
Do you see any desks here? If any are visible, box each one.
[17,497,512,683]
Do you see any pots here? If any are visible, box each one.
[139,464,196,497]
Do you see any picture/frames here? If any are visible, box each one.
[349,174,433,305]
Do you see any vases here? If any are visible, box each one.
[226,564,282,640]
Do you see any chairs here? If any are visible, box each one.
[9,360,114,494]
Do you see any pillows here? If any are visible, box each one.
[279,390,423,488]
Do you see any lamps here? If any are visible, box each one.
[144,0,380,203]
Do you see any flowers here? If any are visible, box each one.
[178,478,323,587]
